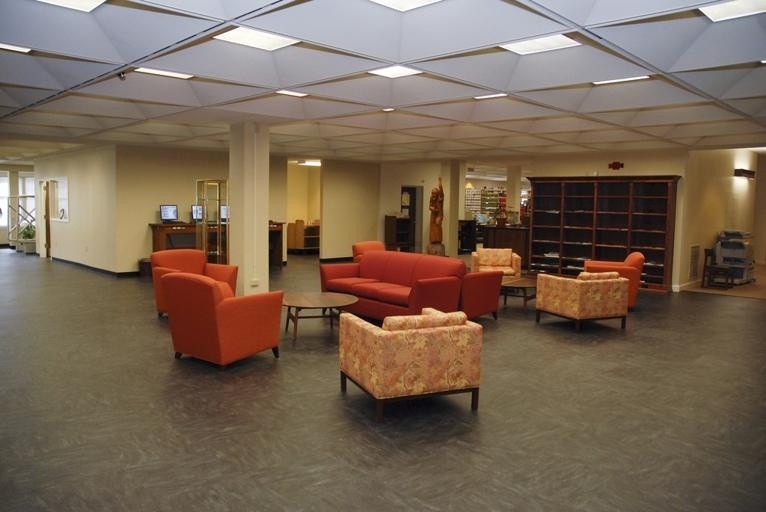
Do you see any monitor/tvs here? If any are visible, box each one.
[160,205,178,224]
[220,205,229,222]
[192,205,203,223]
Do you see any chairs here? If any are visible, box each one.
[157,271,285,371]
[335,302,485,426]
[700,247,735,289]
[148,246,240,322]
[532,270,630,338]
[582,250,646,312]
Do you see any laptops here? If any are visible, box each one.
[476,214,487,225]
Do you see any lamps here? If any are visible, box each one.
[295,160,321,166]
[734,169,755,179]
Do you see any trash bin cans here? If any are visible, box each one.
[138,258,152,277]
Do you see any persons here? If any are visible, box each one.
[429,176,444,245]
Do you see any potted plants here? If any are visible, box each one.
[17,223,36,255]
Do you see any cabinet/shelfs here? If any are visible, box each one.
[523,172,683,297]
[145,218,321,269]
[463,188,508,216]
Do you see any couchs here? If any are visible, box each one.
[316,236,523,328]
